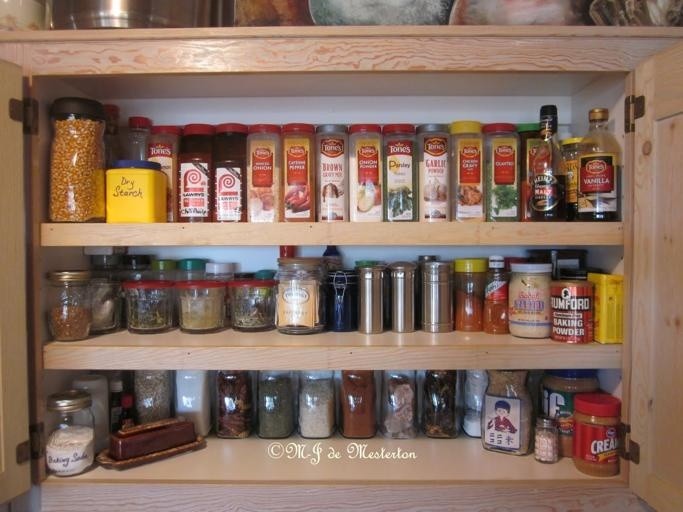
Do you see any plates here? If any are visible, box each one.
[305,0,683,29]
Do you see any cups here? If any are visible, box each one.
[0,1,149,30]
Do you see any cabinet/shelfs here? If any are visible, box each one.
[39,221,632,485]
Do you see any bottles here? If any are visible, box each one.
[45,244,511,341]
[43,93,622,224]
[41,367,559,478]
